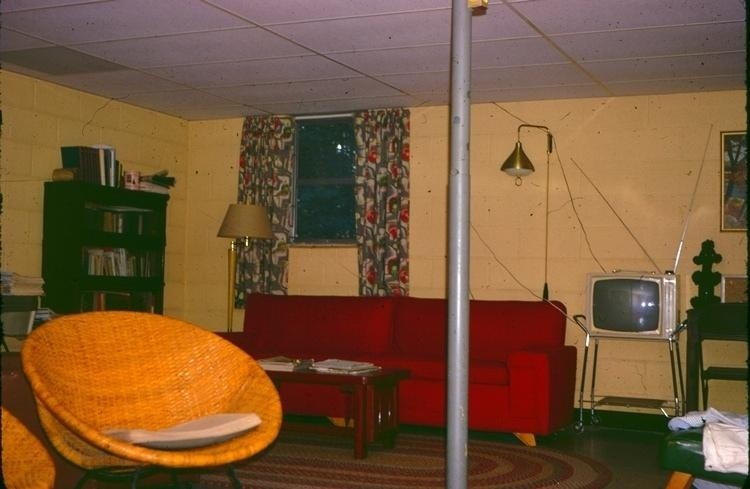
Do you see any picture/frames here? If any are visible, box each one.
[721,275,749,303]
[721,130,749,230]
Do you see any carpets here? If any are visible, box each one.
[202,431,614,489]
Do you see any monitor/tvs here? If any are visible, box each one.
[586,269,677,340]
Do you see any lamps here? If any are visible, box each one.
[502,124,552,301]
[218,203,275,333]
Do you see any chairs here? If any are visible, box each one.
[22,308,282,486]
[657,303,748,487]
[3,406,57,487]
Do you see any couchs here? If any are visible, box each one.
[222,295,575,447]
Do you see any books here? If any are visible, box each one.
[0,269,61,330]
[60,144,176,315]
[256,353,383,376]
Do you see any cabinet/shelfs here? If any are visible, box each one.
[45,181,170,316]
[570,315,685,430]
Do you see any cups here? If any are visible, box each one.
[124,169,141,192]
